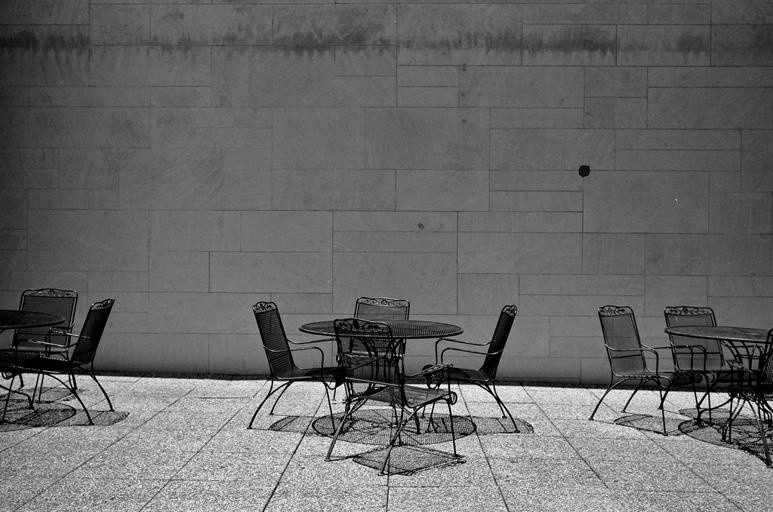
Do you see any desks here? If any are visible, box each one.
[665,325,773,432]
[0,309,66,408]
[298,318,464,435]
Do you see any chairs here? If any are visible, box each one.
[657,306,751,410]
[724,328,773,468]
[10,288,79,402]
[332,296,421,402]
[248,301,354,435]
[0,297,116,425]
[588,305,702,437]
[422,305,534,433]
[324,319,471,477]
